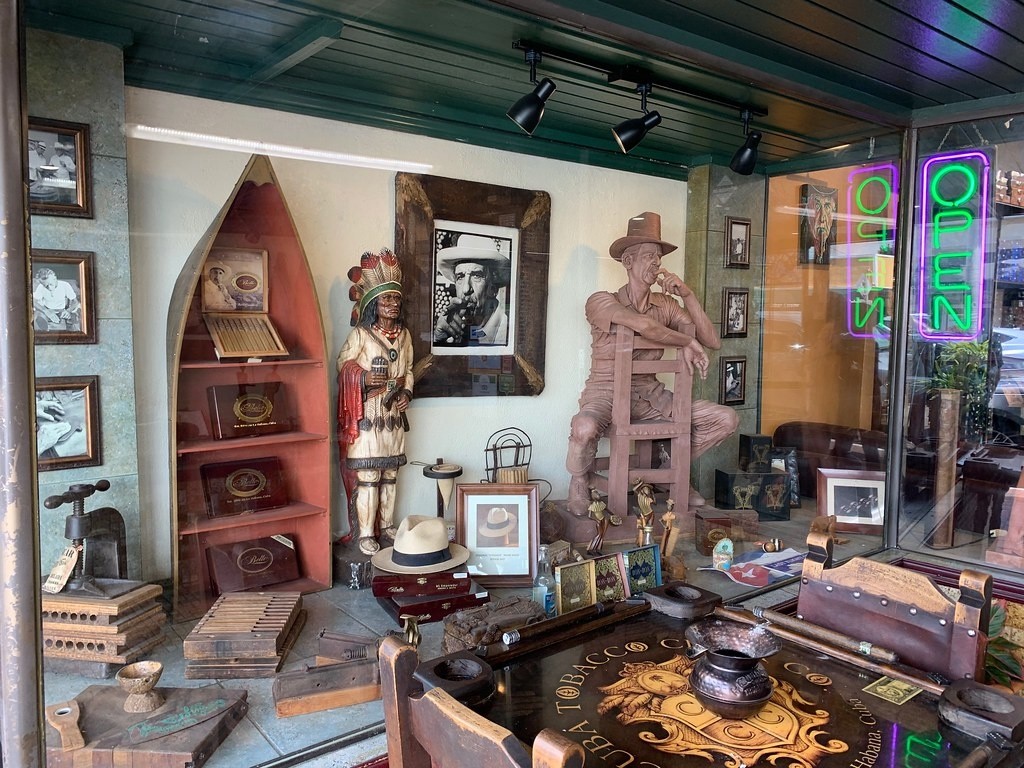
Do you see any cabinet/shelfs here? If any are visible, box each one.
[166,157,341,622]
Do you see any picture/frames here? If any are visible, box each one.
[30,247,97,347]
[816,468,888,535]
[452,481,538,585]
[723,214,754,267]
[717,353,749,405]
[35,376,103,473]
[28,115,92,219]
[393,171,550,398]
[720,285,750,339]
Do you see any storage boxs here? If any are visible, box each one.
[590,551,630,602]
[622,542,661,596]
[695,510,732,556]
[554,560,597,616]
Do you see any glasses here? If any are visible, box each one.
[38,147,45,151]
[211,271,224,275]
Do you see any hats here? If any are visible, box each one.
[727,364,734,371]
[479,508,517,537]
[37,142,47,150]
[371,515,471,574]
[205,261,231,281]
[437,234,511,288]
[54,142,65,148]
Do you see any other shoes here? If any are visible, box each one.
[906,441,917,449]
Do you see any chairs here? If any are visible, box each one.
[586,315,696,516]
[797,534,993,683]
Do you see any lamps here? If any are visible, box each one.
[730,106,765,175]
[611,75,661,154]
[504,50,556,135]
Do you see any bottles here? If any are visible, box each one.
[533,545,557,620]
[643,526,654,546]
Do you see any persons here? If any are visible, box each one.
[734,238,742,262]
[726,363,736,393]
[733,443,784,508]
[36,400,71,455]
[659,443,670,464]
[33,268,78,323]
[28,141,76,204]
[435,234,510,344]
[566,212,739,517]
[337,247,413,555]
[586,479,677,556]
[204,261,236,311]
